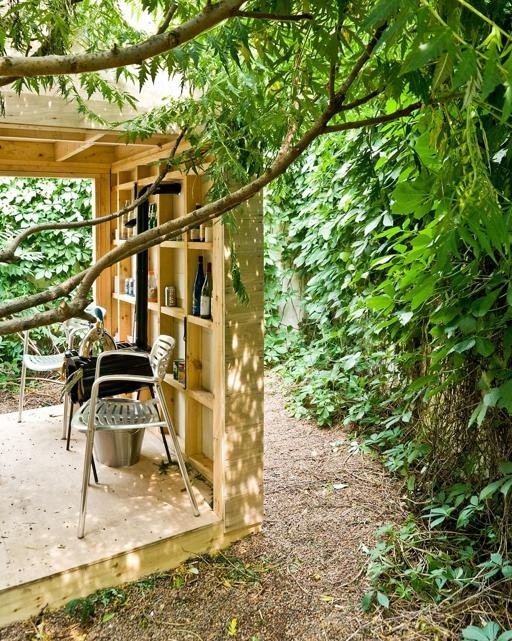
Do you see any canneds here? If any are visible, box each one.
[124,278,133,296]
[164,286,176,308]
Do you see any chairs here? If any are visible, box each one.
[17,305,107,440]
[71,335,200,539]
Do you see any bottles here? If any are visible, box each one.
[189,202,212,245]
[190,253,212,318]
[147,272,158,302]
[119,198,158,245]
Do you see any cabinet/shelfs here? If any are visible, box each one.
[109,137,217,485]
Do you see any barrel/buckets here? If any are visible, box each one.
[92,398,149,467]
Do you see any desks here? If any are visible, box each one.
[65,344,173,483]
